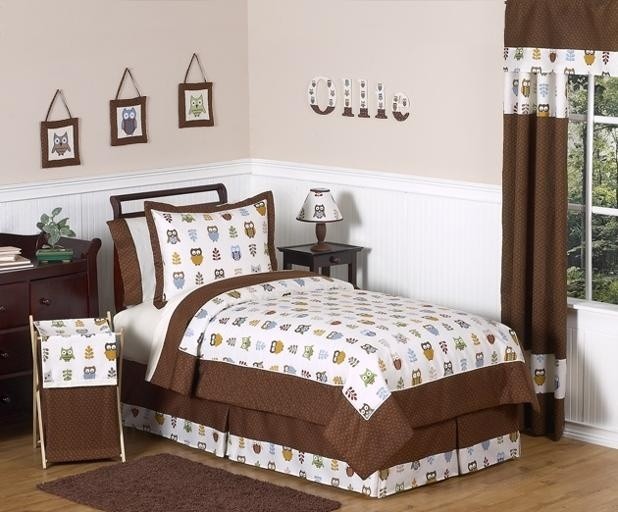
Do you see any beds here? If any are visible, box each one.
[105,183,541,501]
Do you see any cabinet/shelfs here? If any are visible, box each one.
[1,233,103,435]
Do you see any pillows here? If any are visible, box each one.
[143,189,276,309]
[105,216,157,306]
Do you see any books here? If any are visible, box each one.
[0,245,34,274]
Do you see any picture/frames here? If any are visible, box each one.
[41,117,80,168]
[110,96,147,146]
[178,81,214,128]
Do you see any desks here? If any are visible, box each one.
[275,241,364,288]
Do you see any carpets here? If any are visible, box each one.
[37,453,343,511]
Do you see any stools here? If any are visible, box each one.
[27,314,126,470]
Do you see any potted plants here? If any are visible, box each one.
[34,206,77,263]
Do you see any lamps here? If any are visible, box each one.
[295,187,343,252]
